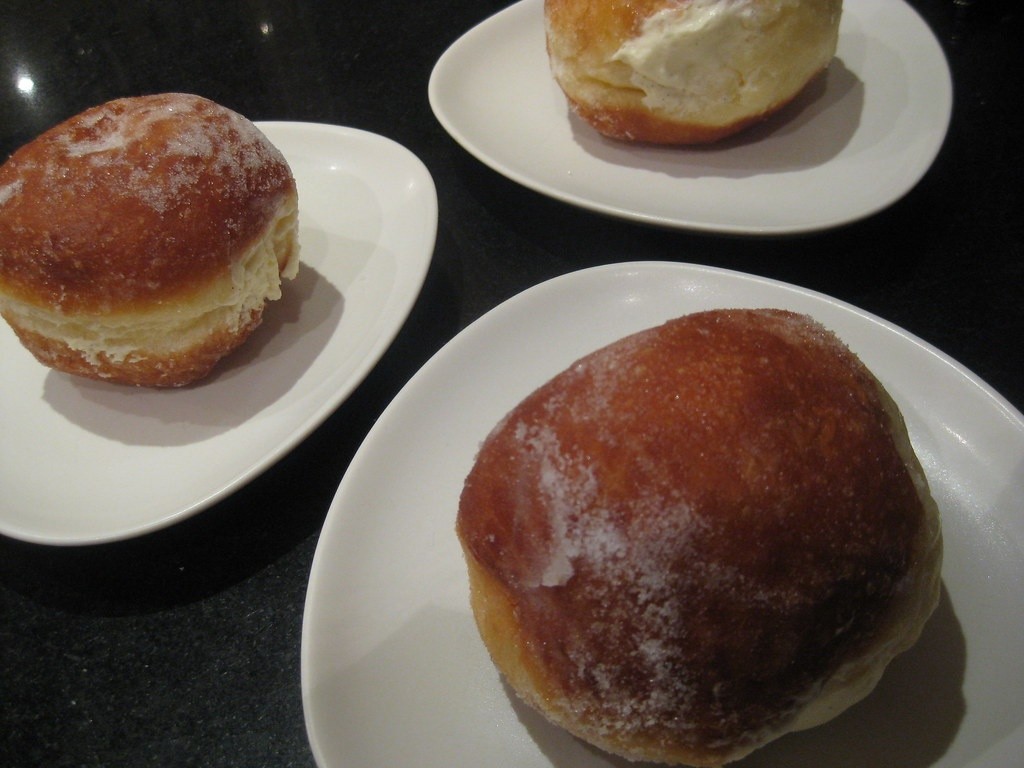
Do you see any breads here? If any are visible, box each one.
[0,94,298,392]
[543,0,844,142]
[457,308,944,768]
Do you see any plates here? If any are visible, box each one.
[0,121,440,550]
[428,0,953,237]
[301,262,1024,768]
